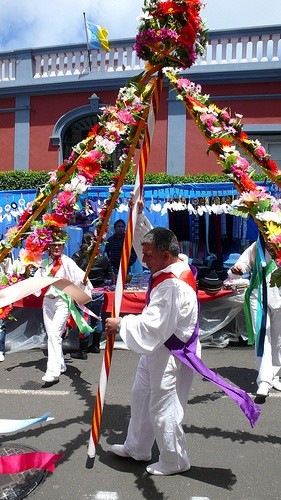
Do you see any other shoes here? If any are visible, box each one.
[0,351,6,362]
[41,376,59,383]
[146,461,191,476]
[273,379,281,390]
[61,368,66,373]
[257,382,270,396]
[111,444,132,457]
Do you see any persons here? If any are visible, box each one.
[0,248,13,362]
[106,228,202,475]
[105,219,137,284]
[228,196,281,404]
[31,230,94,382]
[69,231,110,359]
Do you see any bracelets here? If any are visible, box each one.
[116,320,120,333]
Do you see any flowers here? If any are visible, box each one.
[162,69,281,268]
[0,71,157,290]
[133,0,209,70]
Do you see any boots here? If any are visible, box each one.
[70,336,89,360]
[87,331,102,353]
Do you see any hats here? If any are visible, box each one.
[50,230,67,245]
[224,253,240,264]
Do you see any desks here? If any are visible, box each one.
[13,283,234,313]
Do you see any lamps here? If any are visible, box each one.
[50,137,60,147]
